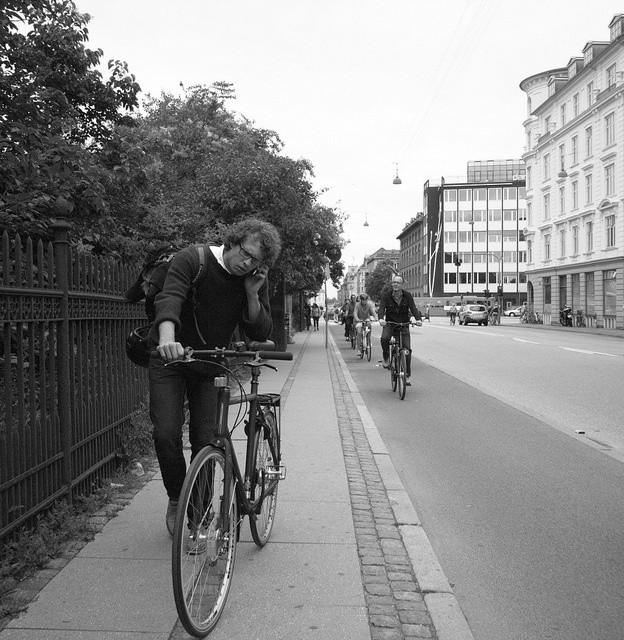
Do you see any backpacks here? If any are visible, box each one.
[124,244,207,368]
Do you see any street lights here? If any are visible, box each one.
[469,219,474,294]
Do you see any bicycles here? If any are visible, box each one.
[356,320,376,362]
[381,321,416,400]
[348,315,356,349]
[152,339,293,638]
[450,315,455,326]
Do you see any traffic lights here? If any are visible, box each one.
[498,286,503,296]
[453,254,458,263]
[459,258,462,266]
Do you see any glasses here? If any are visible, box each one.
[393,281,402,284]
[239,243,265,267]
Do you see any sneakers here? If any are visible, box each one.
[406,378,411,385]
[187,524,207,553]
[167,501,178,536]
[346,337,349,341]
[383,359,389,368]
[357,351,361,355]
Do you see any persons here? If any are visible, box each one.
[356,296,361,302]
[378,275,422,384]
[322,311,324,318]
[340,299,351,325]
[353,294,378,356]
[490,302,501,325]
[422,305,431,322]
[344,294,357,341]
[304,302,311,330]
[332,306,338,323]
[536,312,540,323]
[449,303,457,324]
[312,303,321,331]
[519,303,529,323]
[146,218,282,554]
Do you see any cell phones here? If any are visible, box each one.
[252,269,257,276]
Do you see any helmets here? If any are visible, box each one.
[360,294,368,300]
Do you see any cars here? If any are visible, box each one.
[457,304,489,325]
[324,310,342,321]
[503,305,526,317]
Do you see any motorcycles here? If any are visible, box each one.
[489,306,499,325]
[560,308,572,327]
